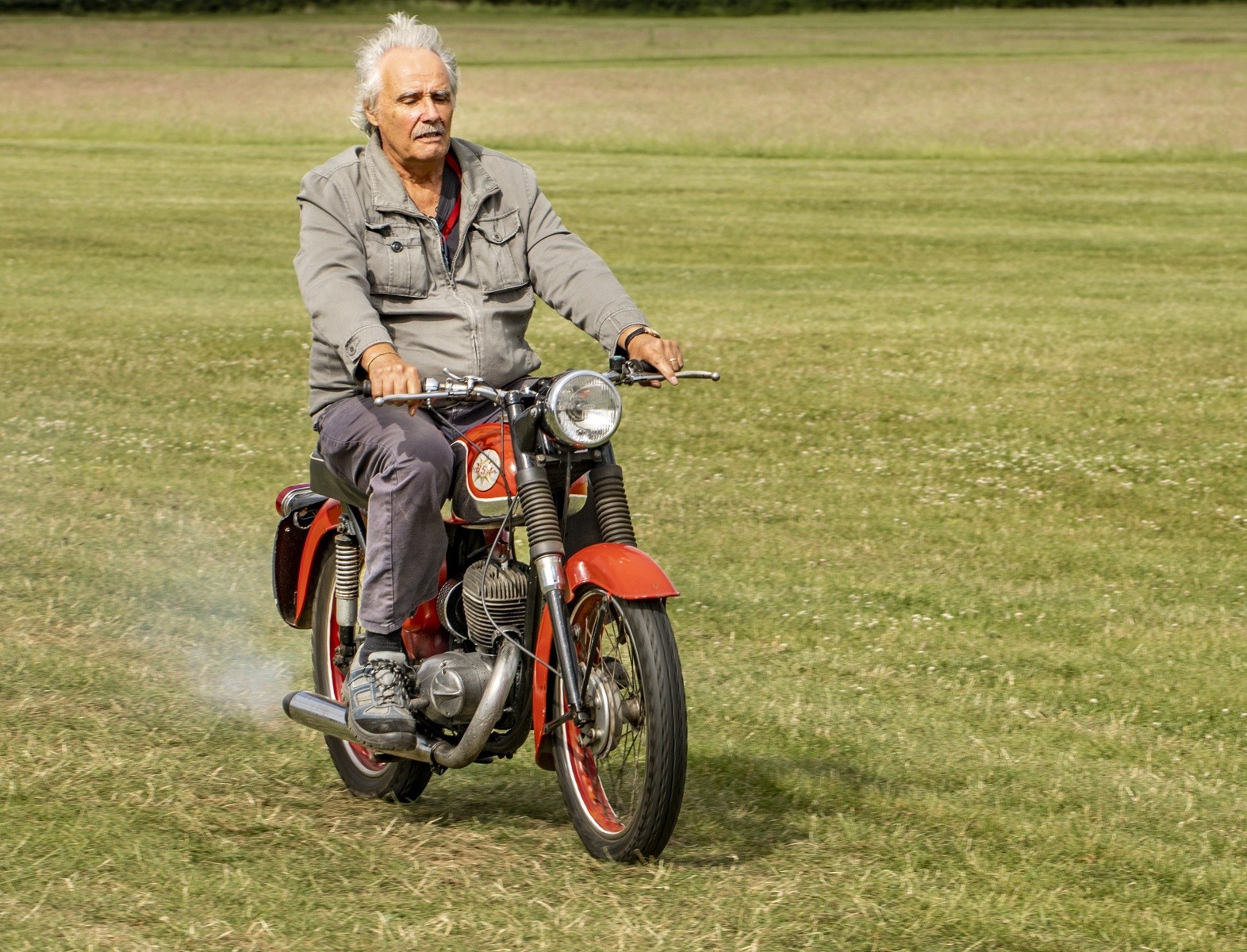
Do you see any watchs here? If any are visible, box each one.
[625,326,660,350]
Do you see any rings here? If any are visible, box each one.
[670,358,678,362]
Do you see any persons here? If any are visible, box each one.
[296,11,684,752]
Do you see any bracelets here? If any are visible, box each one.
[367,352,402,372]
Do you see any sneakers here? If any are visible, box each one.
[340,642,419,752]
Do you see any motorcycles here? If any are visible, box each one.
[271,355,722,865]
